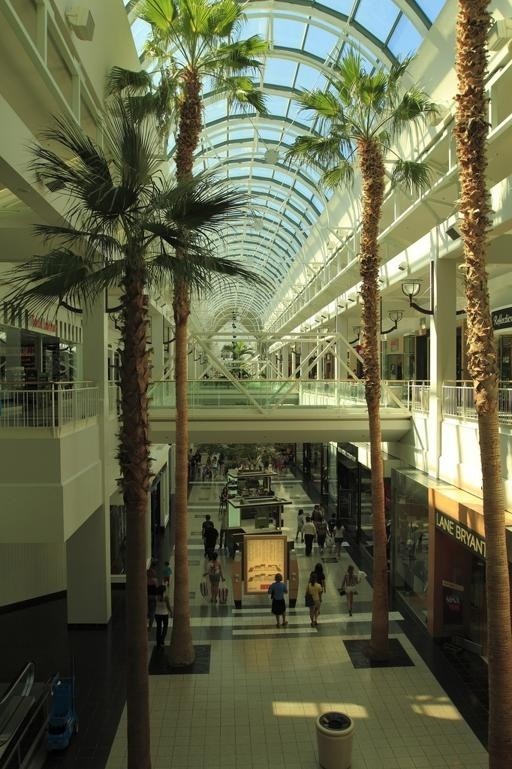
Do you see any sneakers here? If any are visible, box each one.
[349,609,353,617]
[276,621,288,629]
[310,620,318,627]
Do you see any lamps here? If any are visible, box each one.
[381,309,405,335]
[317,326,336,344]
[348,325,362,345]
[401,283,434,316]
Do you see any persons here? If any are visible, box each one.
[309,564,326,615]
[147,569,158,618]
[154,586,173,649]
[161,561,173,585]
[203,552,225,602]
[306,574,322,627]
[188,449,346,560]
[341,565,359,616]
[268,574,288,627]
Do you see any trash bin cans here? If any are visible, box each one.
[316,710,355,769]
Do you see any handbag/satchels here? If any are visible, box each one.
[305,594,315,607]
